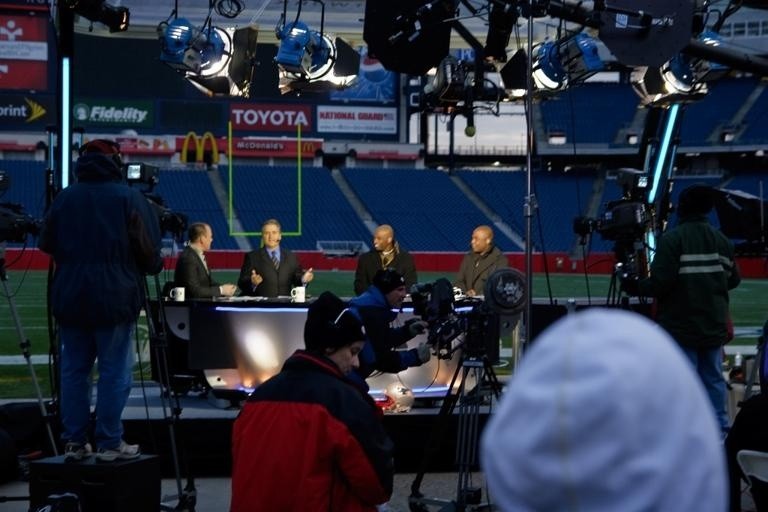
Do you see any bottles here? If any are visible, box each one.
[729,352,745,389]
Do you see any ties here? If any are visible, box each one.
[383,257,389,269]
[271,252,279,272]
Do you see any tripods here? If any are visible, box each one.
[409,368,502,511]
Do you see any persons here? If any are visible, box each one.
[610,178,746,441]
[344,265,439,392]
[36,132,167,464]
[218,216,318,302]
[722,321,767,510]
[170,215,239,303]
[227,290,399,511]
[450,220,513,306]
[351,222,422,296]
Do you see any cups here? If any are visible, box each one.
[291,285,305,304]
[170,286,185,302]
[451,286,463,299]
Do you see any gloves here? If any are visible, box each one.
[400,318,428,341]
[412,343,432,366]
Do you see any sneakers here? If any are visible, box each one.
[96,440,140,461]
[65,442,93,459]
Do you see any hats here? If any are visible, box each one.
[304,291,365,354]
[377,268,406,294]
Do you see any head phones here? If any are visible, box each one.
[114,148,123,164]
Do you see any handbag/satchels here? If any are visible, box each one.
[410,278,455,324]
